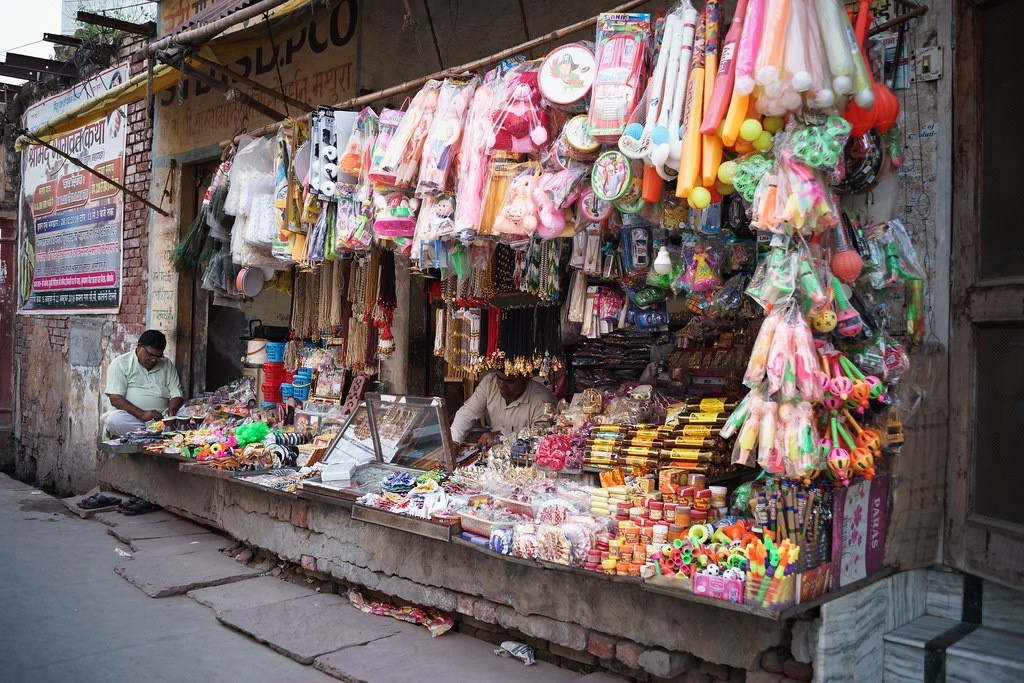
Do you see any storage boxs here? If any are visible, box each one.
[498,495,536,518]
[428,511,461,525]
[458,509,514,538]
[693,562,831,611]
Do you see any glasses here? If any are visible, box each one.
[139,346,164,362]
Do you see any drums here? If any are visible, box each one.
[236,266,265,298]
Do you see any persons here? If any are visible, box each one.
[438,366,558,463]
[384,87,439,189]
[689,243,719,290]
[427,195,455,241]
[411,196,435,260]
[20,220,34,305]
[720,241,828,473]
[458,85,489,173]
[104,71,123,139]
[99,329,184,441]
[420,93,465,187]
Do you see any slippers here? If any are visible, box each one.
[77,492,122,508]
[122,498,161,515]
[115,495,140,513]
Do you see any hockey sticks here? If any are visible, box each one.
[618,8,698,170]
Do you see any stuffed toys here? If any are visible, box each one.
[492,172,566,238]
[486,70,553,149]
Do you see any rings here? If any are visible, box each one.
[153,418,155,420]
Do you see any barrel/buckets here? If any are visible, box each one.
[245,338,268,368]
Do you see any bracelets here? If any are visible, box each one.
[454,442,459,446]
